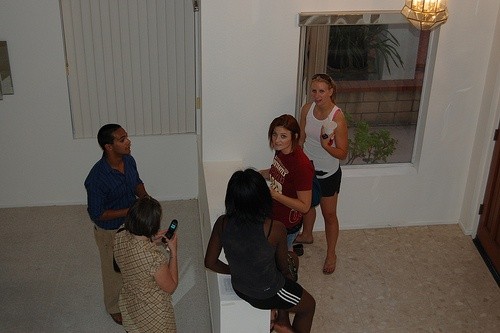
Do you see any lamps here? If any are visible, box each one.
[401,0,448,32]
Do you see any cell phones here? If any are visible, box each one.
[161,219,178,243]
[322,134,334,146]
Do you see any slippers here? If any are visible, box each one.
[270,311,277,332]
[323,252,337,274]
[292,233,314,243]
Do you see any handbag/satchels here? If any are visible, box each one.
[310,159,322,209]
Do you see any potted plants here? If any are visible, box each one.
[327,24,406,78]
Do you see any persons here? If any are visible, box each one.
[84,124,149,325]
[112,195,178,333]
[292,74,349,275]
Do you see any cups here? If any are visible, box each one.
[323,120,337,139]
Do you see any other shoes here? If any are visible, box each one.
[111,312,122,325]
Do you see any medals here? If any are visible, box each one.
[204,114,316,333]
